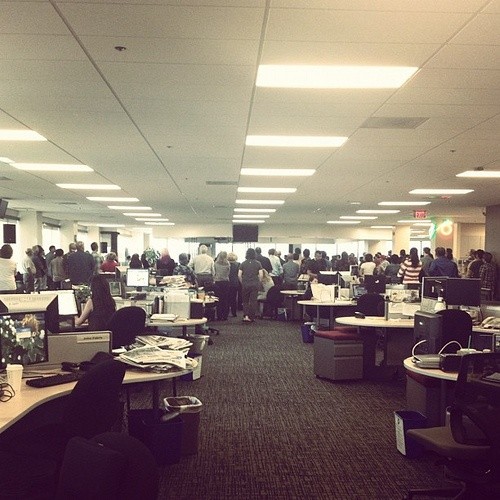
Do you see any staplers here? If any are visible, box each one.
[61,361,79,373]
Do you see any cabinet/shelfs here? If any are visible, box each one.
[314,326,364,381]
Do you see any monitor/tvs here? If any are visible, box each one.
[115,266,149,292]
[352,284,367,299]
[414,309,443,354]
[422,276,481,308]
[0,288,78,363]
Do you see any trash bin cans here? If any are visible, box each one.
[300,324,315,344]
[163,395,203,455]
[178,333,209,378]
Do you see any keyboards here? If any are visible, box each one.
[24,370,88,388]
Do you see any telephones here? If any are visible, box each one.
[480,316,500,329]
[341,295,349,301]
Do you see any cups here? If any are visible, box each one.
[6,364,24,393]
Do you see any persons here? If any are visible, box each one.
[22,241,119,295]
[238,248,263,322]
[127,244,497,321]
[67,274,116,331]
[0,245,18,290]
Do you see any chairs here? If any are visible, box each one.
[404,353,500,500]
[27,359,126,500]
[105,304,146,349]
[348,294,385,338]
[434,309,472,352]
[195,282,220,345]
[257,286,283,321]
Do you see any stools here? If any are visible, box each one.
[405,368,440,426]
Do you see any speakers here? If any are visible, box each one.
[3,224,16,243]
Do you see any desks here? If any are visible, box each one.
[0,357,198,434]
[335,316,500,383]
[280,289,307,321]
[59,317,207,340]
[147,295,218,304]
[296,299,358,331]
[403,356,500,468]
